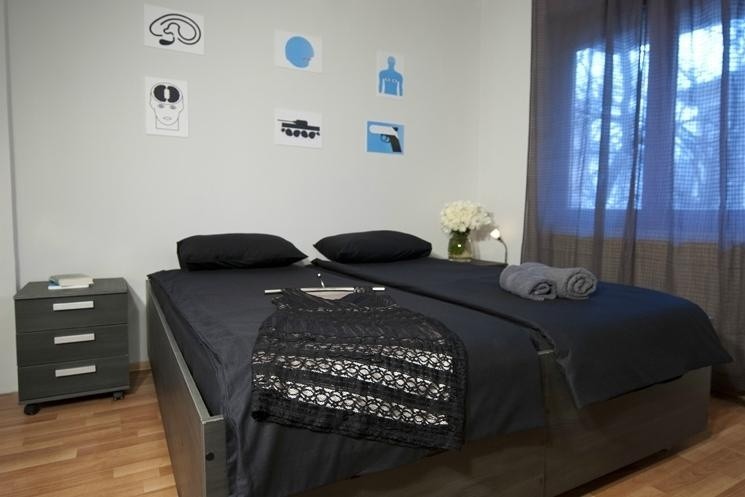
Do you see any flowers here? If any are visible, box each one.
[440,199,492,236]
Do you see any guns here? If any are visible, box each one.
[368,124,401,153]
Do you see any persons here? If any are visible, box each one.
[149,81,184,130]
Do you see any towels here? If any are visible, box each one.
[498,261,599,301]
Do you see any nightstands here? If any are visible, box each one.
[12,273,130,416]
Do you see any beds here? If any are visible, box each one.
[148,255,732,497]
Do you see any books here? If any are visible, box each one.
[50,274,94,286]
[47,281,89,290]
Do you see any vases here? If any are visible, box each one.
[447,231,474,264]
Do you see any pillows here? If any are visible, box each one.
[313,230,433,263]
[176,233,308,270]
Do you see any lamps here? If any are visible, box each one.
[489,227,508,264]
